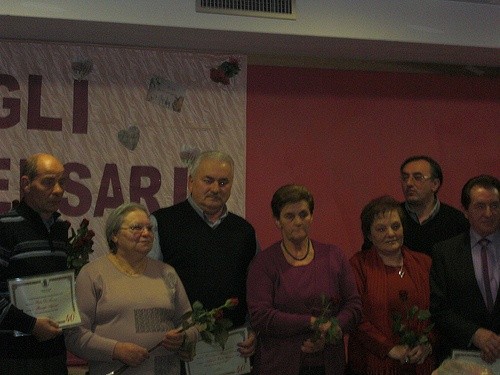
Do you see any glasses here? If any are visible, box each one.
[396,175,434,185]
[118,223,157,234]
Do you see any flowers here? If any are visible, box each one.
[105,298,239,374]
[391,288,437,367]
[304,296,347,372]
[60,219,94,276]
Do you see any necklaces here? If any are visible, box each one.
[399,257,405,278]
[114,257,146,275]
[283,237,310,261]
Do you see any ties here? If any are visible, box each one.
[478,239,492,309]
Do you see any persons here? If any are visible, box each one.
[345,194,440,375]
[245,183,363,375]
[145,149,258,375]
[0,151,70,375]
[64,202,200,375]
[361,154,469,256]
[428,174,500,365]
